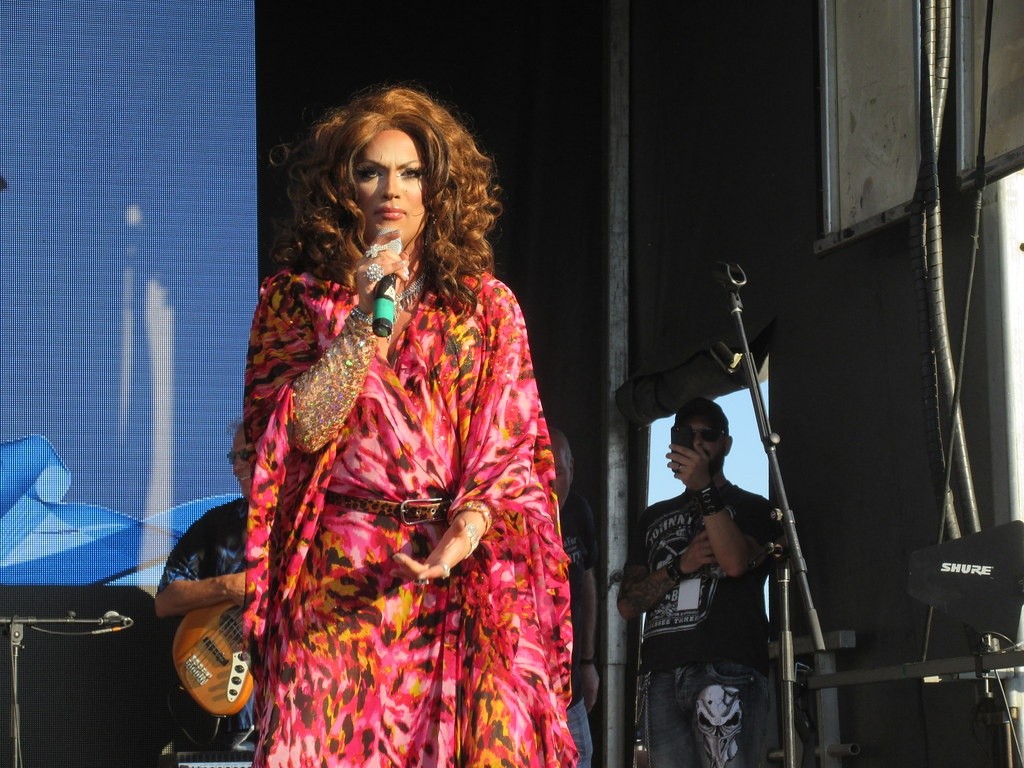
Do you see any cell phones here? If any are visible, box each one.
[671,425,694,473]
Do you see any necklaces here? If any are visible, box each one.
[392,271,425,324]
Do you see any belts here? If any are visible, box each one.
[323,489,455,527]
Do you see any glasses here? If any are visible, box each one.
[226,449,253,462]
[693,425,725,441]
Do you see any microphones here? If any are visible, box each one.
[371,227,402,337]
[103,611,134,629]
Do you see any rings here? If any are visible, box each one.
[677,463,682,472]
[416,564,430,584]
[365,243,388,259]
[364,263,384,283]
[441,563,450,580]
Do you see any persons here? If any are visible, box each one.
[617,395,790,768]
[154,416,258,745]
[241,85,598,768]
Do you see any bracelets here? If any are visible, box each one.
[574,658,595,664]
[349,307,375,326]
[667,554,687,582]
[696,480,726,516]
[466,523,479,560]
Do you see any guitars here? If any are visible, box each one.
[169,597,258,718]
